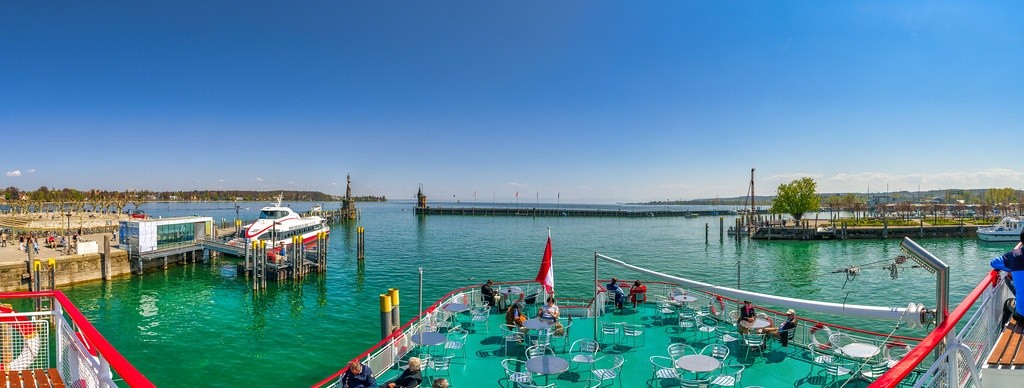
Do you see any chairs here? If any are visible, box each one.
[398,286,912,388]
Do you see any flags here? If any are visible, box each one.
[534,236,556,295]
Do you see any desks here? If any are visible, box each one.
[739,318,771,356]
[443,304,470,332]
[673,295,698,323]
[521,318,550,353]
[499,287,522,312]
[676,355,720,383]
[842,343,881,383]
[526,356,569,386]
[411,332,447,368]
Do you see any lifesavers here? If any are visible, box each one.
[453,293,468,306]
[393,333,408,359]
[809,323,832,350]
[709,296,725,319]
[755,313,773,327]
[427,306,436,330]
[883,341,913,360]
[671,287,686,307]
[504,285,524,305]
[69,332,101,388]
[0,306,40,370]
[618,284,632,300]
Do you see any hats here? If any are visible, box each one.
[744,299,752,303]
[405,377,422,388]
[786,309,796,315]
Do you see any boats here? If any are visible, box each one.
[685,212,702,219]
[560,213,568,216]
[728,208,757,215]
[311,204,322,212]
[245,201,330,256]
[977,215,1024,241]
[706,212,720,216]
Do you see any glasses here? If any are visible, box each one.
[787,314,790,316]
[747,302,751,304]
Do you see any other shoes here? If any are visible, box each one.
[757,345,767,351]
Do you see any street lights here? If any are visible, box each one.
[65,214,73,255]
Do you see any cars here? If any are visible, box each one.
[131,211,148,218]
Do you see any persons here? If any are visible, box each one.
[431,377,450,388]
[505,301,528,344]
[989,225,1024,328]
[18,231,81,256]
[480,278,508,316]
[627,279,647,310]
[341,362,378,388]
[381,357,423,388]
[737,300,758,348]
[1,232,7,248]
[759,309,798,352]
[110,227,117,242]
[537,296,564,338]
[606,277,626,311]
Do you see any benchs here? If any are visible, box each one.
[981,313,1024,388]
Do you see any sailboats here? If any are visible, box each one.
[727,168,762,235]
